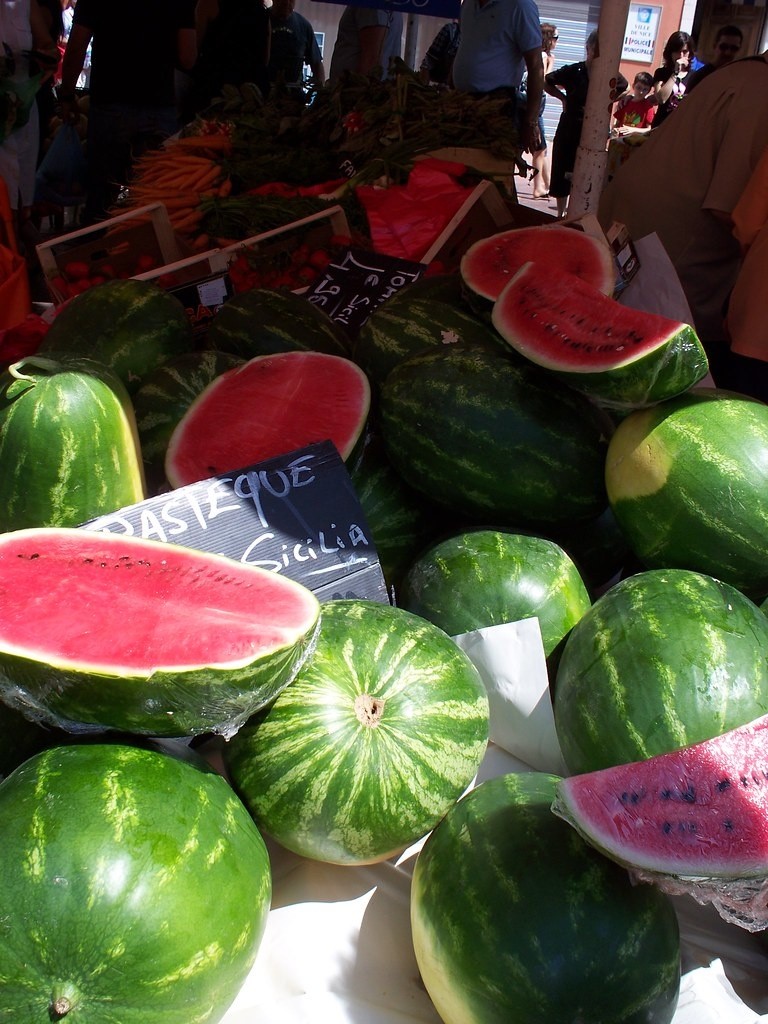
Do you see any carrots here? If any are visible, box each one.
[106,134,233,233]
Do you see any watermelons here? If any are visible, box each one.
[456,223,710,411]
[0,276,768,1024]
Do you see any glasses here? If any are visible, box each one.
[719,43,742,52]
[550,35,559,40]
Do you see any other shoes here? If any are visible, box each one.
[535,191,552,199]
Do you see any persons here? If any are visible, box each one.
[685,25,743,95]
[329,5,403,94]
[516,20,560,201]
[60,0,197,241]
[0,1,93,304]
[616,40,706,113]
[596,51,768,405]
[195,0,272,116]
[609,71,656,140]
[652,31,697,139]
[417,15,462,89]
[265,0,326,113]
[543,29,628,221]
[450,0,545,157]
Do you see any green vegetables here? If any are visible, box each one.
[187,63,518,183]
[0,41,56,143]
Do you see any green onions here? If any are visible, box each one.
[319,136,433,201]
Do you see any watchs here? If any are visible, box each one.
[525,118,539,126]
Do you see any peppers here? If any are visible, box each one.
[226,236,352,293]
